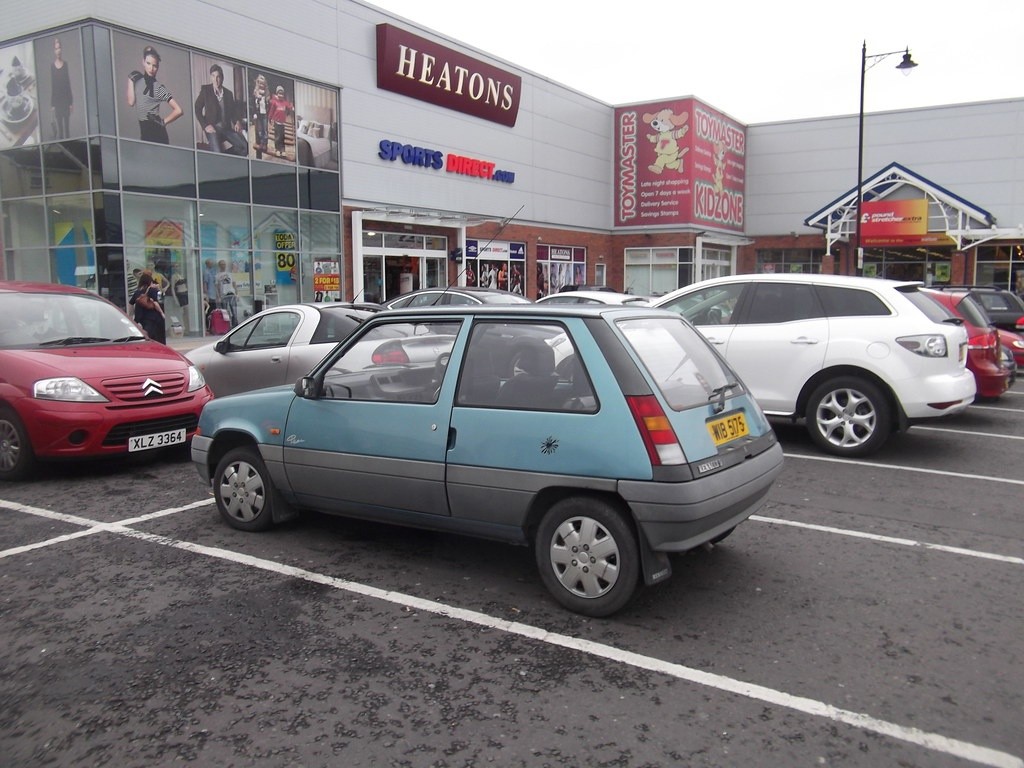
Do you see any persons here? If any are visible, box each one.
[251,73,297,157]
[127,268,167,346]
[467,260,523,295]
[194,64,249,156]
[127,45,183,145]
[536,261,584,296]
[6,39,75,144]
[203,257,241,335]
[125,259,191,335]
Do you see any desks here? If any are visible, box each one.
[0,68,38,148]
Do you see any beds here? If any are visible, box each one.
[297,105,333,169]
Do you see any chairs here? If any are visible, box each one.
[432,350,500,404]
[500,345,559,407]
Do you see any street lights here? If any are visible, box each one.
[853,38,919,275]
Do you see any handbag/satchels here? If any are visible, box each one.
[136,292,157,309]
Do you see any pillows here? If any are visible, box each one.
[299,120,324,138]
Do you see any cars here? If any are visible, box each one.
[0,279,216,483]
[544,271,977,459]
[917,285,1024,404]
[185,301,459,399]
[533,284,684,314]
[190,303,785,619]
[375,286,537,311]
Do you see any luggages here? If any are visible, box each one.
[209,308,228,333]
